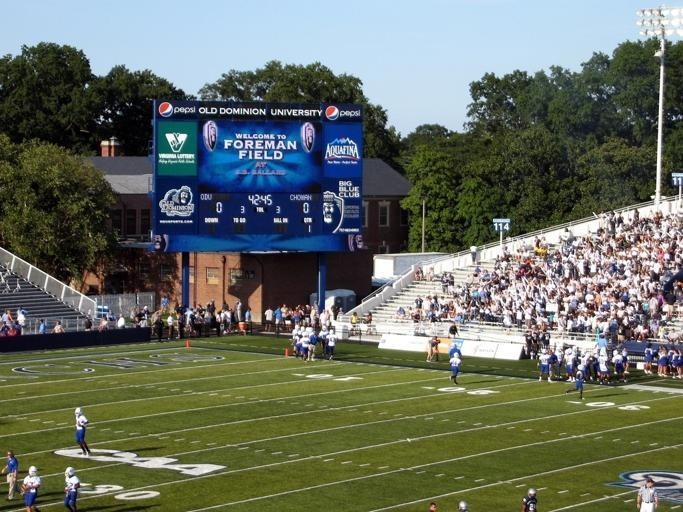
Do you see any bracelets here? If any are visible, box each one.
[636,502,640,503]
[70,486,74,489]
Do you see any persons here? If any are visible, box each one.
[74,407,90,455]
[428,503,436,512]
[21,465,42,511]
[63,466,80,511]
[0,450,21,502]
[264,302,344,360]
[635,478,658,512]
[520,488,537,512]
[84,296,252,343]
[348,205,683,400]
[1,306,65,336]
[457,500,469,512]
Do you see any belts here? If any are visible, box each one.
[643,501,653,503]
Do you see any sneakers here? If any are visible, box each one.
[450,376,452,381]
[644,369,683,379]
[454,382,457,384]
[566,376,631,385]
[539,376,562,383]
[292,351,316,361]
[624,371,630,374]
[82,451,90,456]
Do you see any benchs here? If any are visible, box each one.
[0,266,87,329]
[362,252,494,321]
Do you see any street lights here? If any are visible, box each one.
[634,1,683,206]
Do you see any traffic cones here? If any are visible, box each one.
[282,347,288,359]
[184,339,191,350]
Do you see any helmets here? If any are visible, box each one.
[458,501,467,510]
[577,364,585,371]
[329,329,333,334]
[600,350,606,356]
[322,326,326,331]
[454,352,459,358]
[527,487,537,498]
[29,466,36,476]
[75,407,82,416]
[565,349,572,355]
[542,349,546,354]
[65,467,74,477]
[294,324,312,337]
[613,350,618,356]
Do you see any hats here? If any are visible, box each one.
[647,479,654,483]
